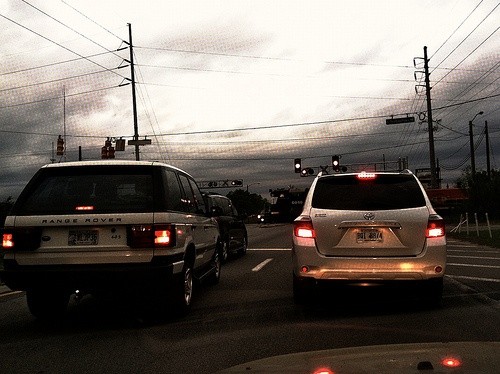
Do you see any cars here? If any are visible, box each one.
[203,192,249,262]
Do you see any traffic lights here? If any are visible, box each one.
[331,154,339,171]
[294,157,301,174]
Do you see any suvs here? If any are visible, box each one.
[291,169,446,296]
[0,159,221,314]
